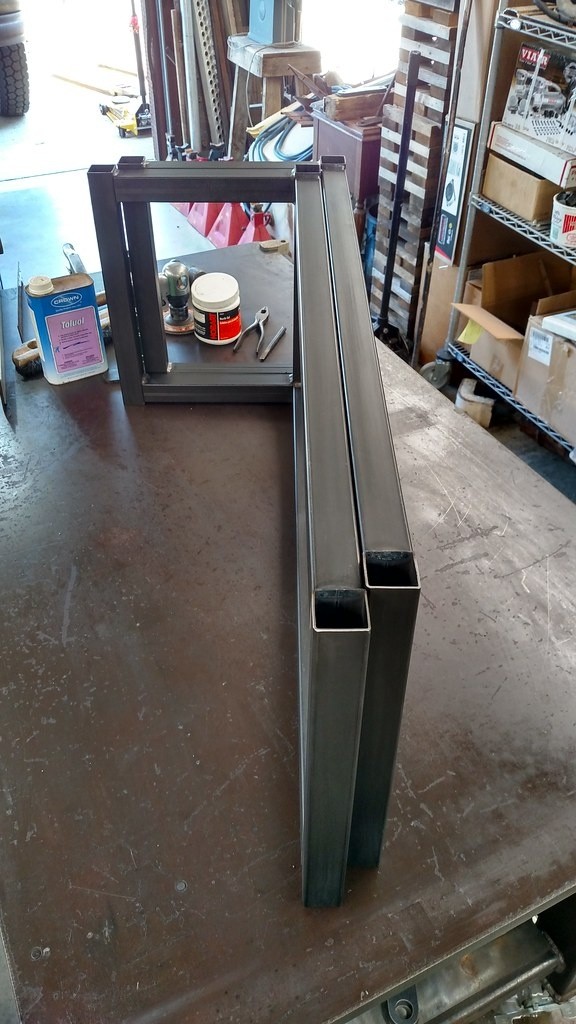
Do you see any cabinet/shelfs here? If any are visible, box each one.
[419,0,576,464]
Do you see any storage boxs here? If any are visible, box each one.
[501,41,576,156]
[482,151,564,223]
[486,120,576,188]
[453,249,576,450]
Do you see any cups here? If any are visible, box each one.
[550,191,576,248]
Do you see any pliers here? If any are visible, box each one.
[233,305,270,354]
